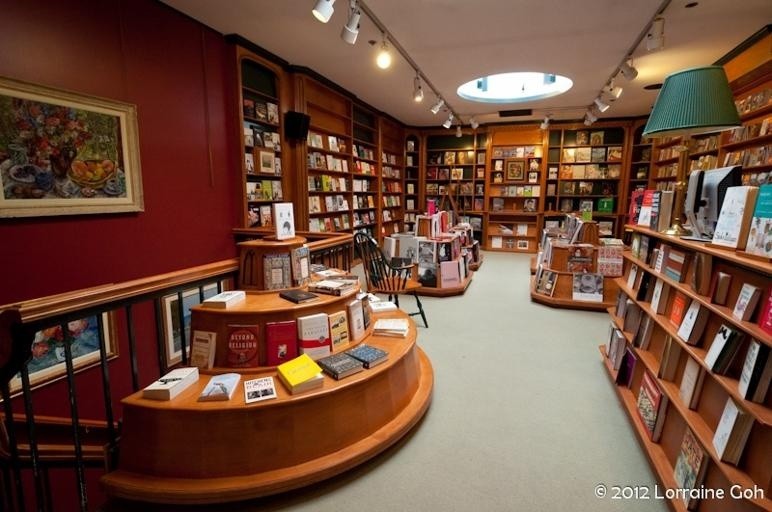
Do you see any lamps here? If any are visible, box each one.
[312,0,464,138]
[583,0,698,128]
[469,114,479,130]
[641,66,741,234]
[540,116,551,130]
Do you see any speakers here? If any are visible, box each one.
[284,110,311,141]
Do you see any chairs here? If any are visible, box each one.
[354,233,428,328]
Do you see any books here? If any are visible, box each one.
[222,323,260,368]
[142,366,201,401]
[196,372,241,403]
[189,330,217,371]
[203,289,246,309]
[242,87,772,512]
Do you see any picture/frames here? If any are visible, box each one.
[0,76,146,219]
[157,277,230,369]
[0,282,121,405]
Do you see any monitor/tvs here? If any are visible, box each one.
[681,165,743,241]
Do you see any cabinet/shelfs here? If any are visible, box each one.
[404,126,423,230]
[649,61,772,192]
[530,211,628,309]
[422,121,629,251]
[621,118,653,239]
[381,208,483,297]
[236,40,403,268]
[598,195,772,512]
